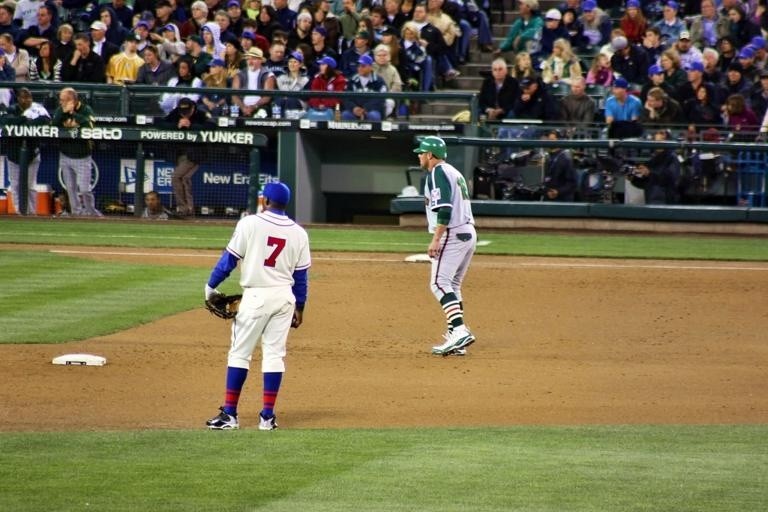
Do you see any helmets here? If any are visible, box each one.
[413,137,447,159]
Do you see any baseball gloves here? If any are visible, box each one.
[207,293,241,318]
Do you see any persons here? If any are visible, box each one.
[480,0,768,207]
[412,136,478,356]
[0,0,494,222]
[204,183,310,431]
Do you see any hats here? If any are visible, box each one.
[258,182,290,204]
[317,57,337,69]
[520,0,764,87]
[90,21,107,31]
[201,1,266,68]
[357,55,373,65]
[179,98,192,114]
[137,21,174,31]
[288,51,302,63]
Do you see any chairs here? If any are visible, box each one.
[406,0,505,100]
[546,49,614,112]
[29,82,168,120]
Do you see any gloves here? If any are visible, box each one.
[206,284,220,300]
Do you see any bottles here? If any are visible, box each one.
[686,119,696,141]
[333,102,342,120]
[270,97,282,120]
[220,100,241,118]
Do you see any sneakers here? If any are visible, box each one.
[258,412,278,429]
[442,68,459,82]
[482,43,492,51]
[432,329,475,355]
[206,406,239,429]
